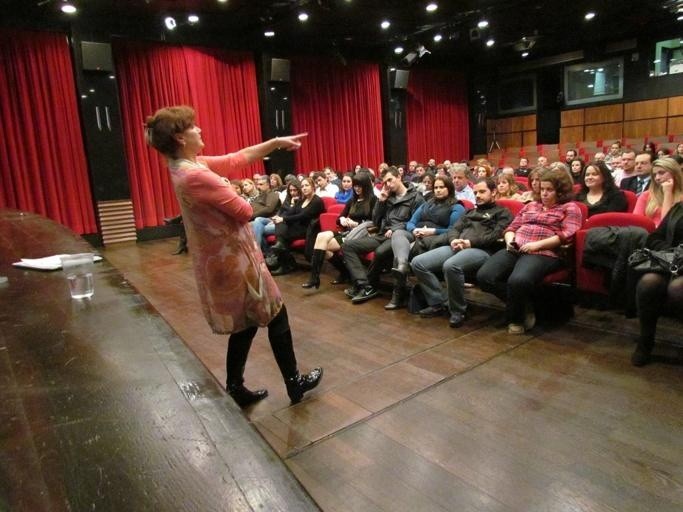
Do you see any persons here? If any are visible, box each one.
[160,214,187,255]
[140,106,321,412]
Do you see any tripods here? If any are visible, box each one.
[489,131,500,152]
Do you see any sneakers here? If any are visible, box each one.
[344,286,358,298]
[524,311,537,331]
[631,335,656,367]
[509,322,523,335]
[419,303,446,319]
[448,311,464,329]
[351,286,378,303]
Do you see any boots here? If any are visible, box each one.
[328,253,349,284]
[302,249,327,289]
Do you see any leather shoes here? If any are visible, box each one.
[271,241,287,252]
[271,266,287,276]
[285,365,323,404]
[224,384,269,408]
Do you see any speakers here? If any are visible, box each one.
[268,58,290,83]
[389,70,410,89]
[77,41,112,76]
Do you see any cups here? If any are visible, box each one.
[58,253,94,300]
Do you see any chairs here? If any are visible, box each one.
[467,136,681,170]
[542,184,658,304]
[321,196,345,232]
[495,199,526,217]
[514,177,530,190]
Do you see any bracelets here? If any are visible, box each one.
[274,135,281,150]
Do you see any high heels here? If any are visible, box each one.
[385,293,403,310]
[391,266,407,288]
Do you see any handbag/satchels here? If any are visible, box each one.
[626,243,683,274]
